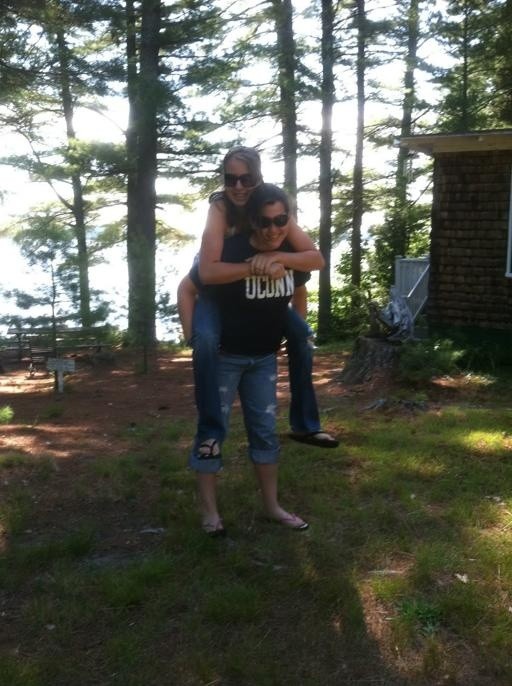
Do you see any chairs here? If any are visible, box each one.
[7,327,110,379]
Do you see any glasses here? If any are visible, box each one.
[258,215,290,227]
[224,173,258,188]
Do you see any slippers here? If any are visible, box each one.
[268,514,310,531]
[202,518,225,537]
[194,440,221,460]
[288,429,339,448]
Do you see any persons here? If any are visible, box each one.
[175,184,311,534]
[193,147,340,462]
